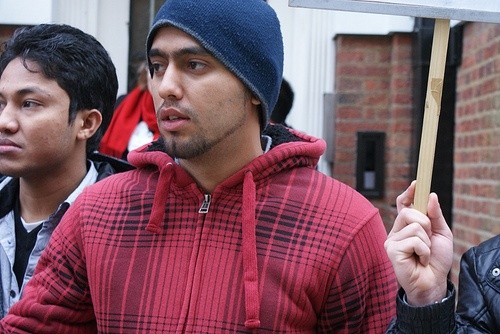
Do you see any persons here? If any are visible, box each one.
[0,0,398,334]
[382,180,500,334]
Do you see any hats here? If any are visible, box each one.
[145,0,284,134]
